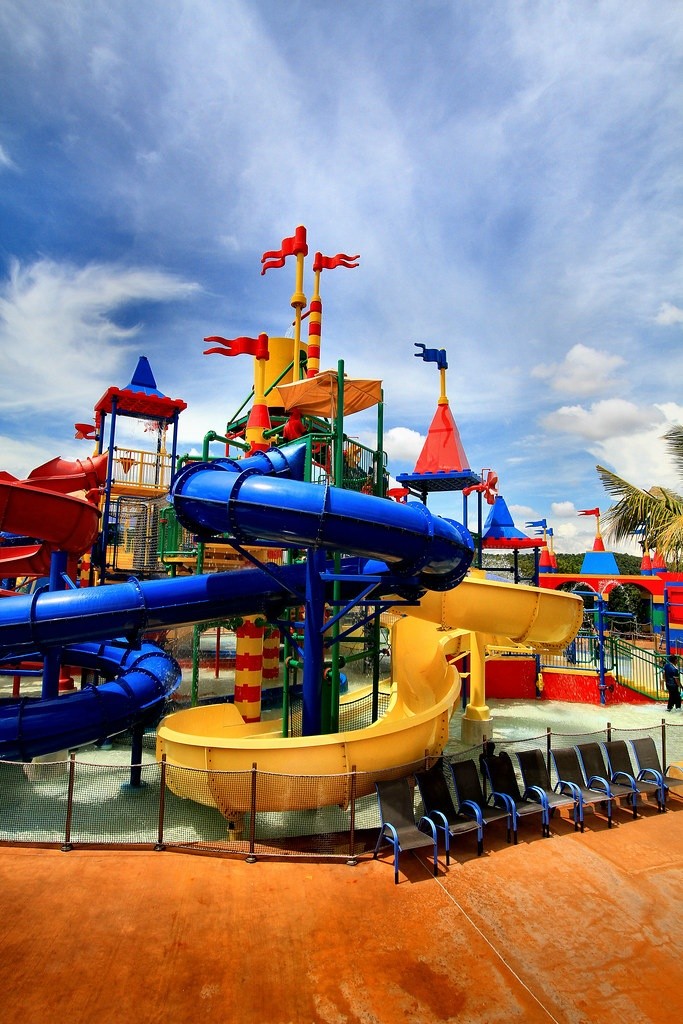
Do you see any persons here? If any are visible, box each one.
[663,655,683,712]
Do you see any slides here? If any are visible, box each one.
[0,440,584,812]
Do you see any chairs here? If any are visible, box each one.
[371,777,438,884]
[575,742,636,822]
[628,738,683,812]
[549,747,611,832]
[414,766,483,866]
[481,751,546,845]
[515,749,578,837]
[601,741,662,816]
[449,759,511,852]
